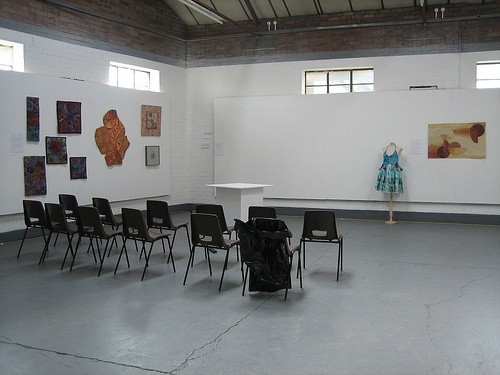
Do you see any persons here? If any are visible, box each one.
[376,141,404,193]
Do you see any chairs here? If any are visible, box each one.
[16,194,343,300]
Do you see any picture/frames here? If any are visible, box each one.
[45,136,67,164]
[70,157,87,179]
[27,96,39,142]
[145,146,160,166]
[56,101,81,134]
[24,156,47,195]
[141,105,161,137]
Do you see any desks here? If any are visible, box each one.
[203,182,274,228]
